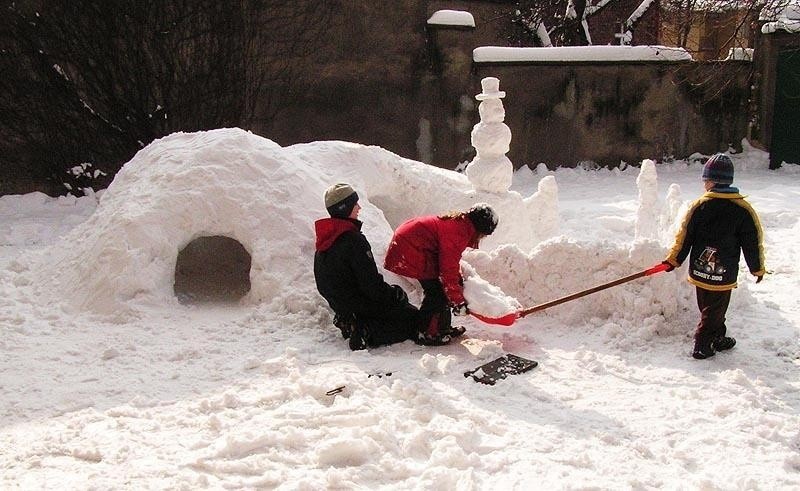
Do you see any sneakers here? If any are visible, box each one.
[415,326,466,345]
[692,337,736,359]
[333,301,379,350]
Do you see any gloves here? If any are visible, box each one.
[452,303,470,316]
[391,284,408,307]
[662,260,675,272]
[756,276,763,283]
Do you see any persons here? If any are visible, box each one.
[382,202,499,345]
[661,153,765,357]
[315,181,429,350]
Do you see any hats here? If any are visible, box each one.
[468,203,499,235]
[701,154,734,184]
[324,183,359,217]
